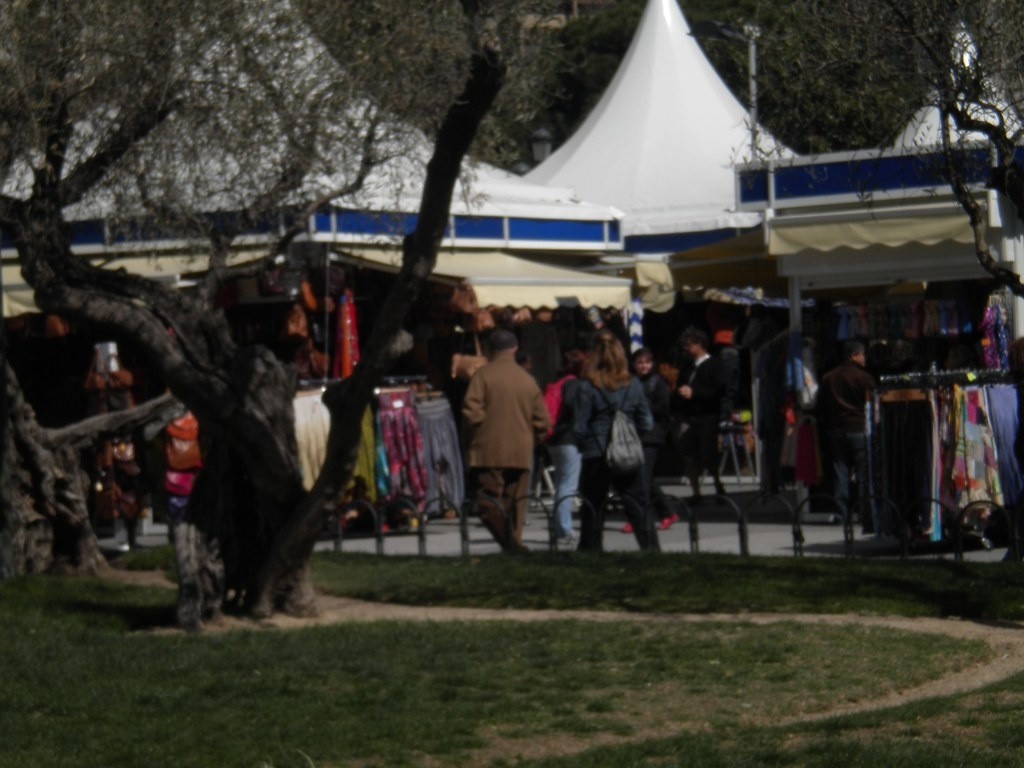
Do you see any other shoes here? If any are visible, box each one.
[657,514,681,529]
[621,523,634,533]
[716,491,726,505]
[687,495,703,507]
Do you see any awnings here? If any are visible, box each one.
[0,250,270,318]
[335,247,632,313]
[667,197,989,288]
[578,260,674,288]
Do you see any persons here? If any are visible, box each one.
[460,328,550,553]
[540,349,589,544]
[571,329,662,552]
[622,348,678,533]
[447,329,750,493]
[680,325,728,506]
[817,341,878,532]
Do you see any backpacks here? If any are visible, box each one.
[587,376,646,470]
